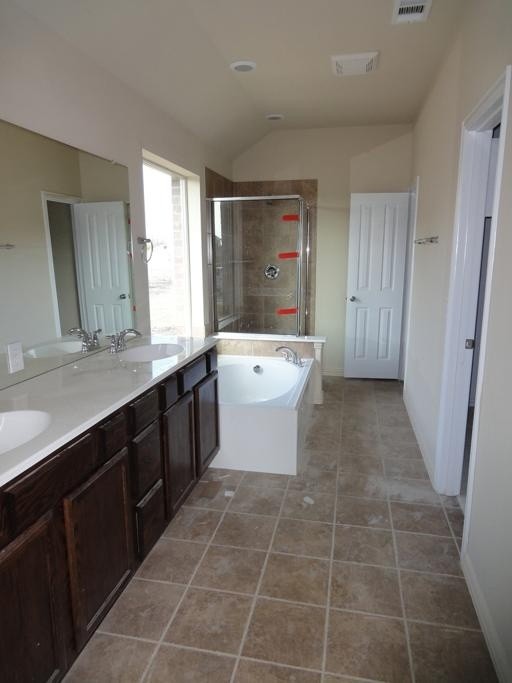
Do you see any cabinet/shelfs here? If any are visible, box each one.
[126,389,169,560]
[165,360,196,521]
[197,344,234,479]
[0,446,63,682]
[64,399,135,655]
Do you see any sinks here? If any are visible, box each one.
[0,409,54,454]
[22,342,82,357]
[114,343,184,363]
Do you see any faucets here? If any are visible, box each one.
[119,329,141,345]
[67,327,90,343]
[275,346,297,362]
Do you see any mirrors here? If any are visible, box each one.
[0,118,138,391]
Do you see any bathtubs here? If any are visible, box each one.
[208,355,315,476]
